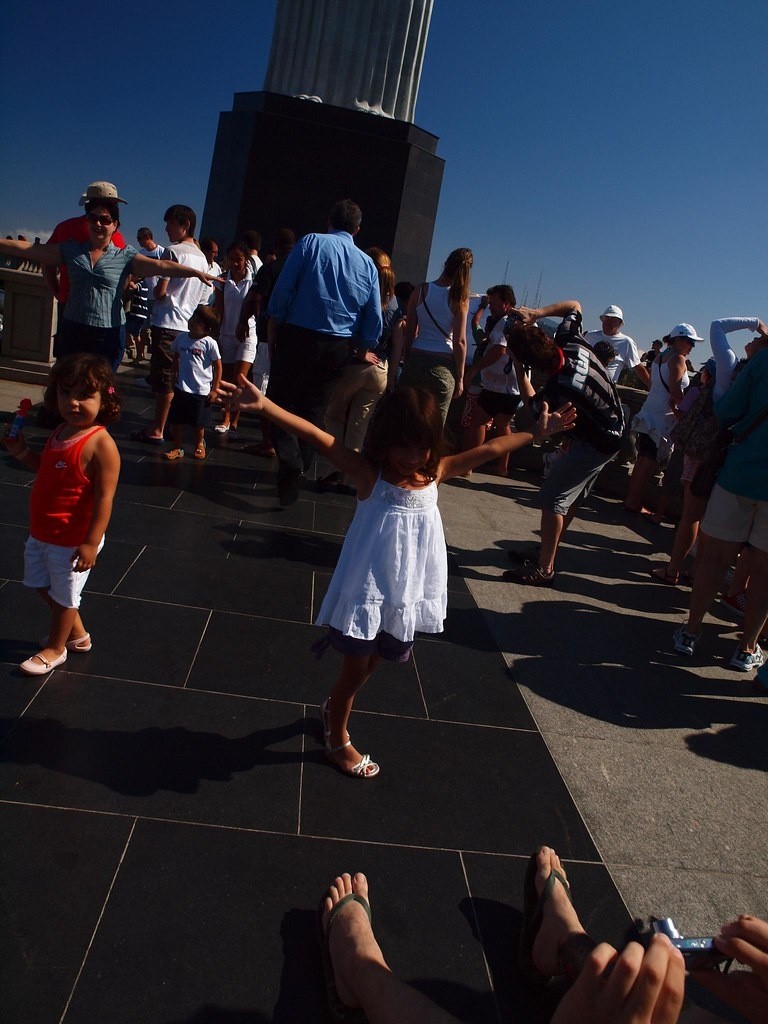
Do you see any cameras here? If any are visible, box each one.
[503,313,523,337]
[630,918,733,972]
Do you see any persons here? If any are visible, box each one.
[317,842,768,1024]
[17,356,122,676]
[654,318,767,585]
[673,348,768,672]
[457,284,719,521]
[0,197,231,379]
[505,303,626,589]
[399,246,474,435]
[722,543,753,619]
[217,372,576,781]
[5,180,422,440]
[311,246,403,497]
[265,200,385,511]
[153,304,224,460]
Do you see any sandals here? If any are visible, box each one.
[324,731,380,778]
[651,565,679,586]
[38,633,93,652]
[193,438,206,460]
[20,645,68,676]
[319,697,350,748]
[164,447,184,460]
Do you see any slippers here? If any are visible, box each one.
[520,845,575,991]
[321,880,380,1023]
[130,429,164,445]
[621,502,656,518]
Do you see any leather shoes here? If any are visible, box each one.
[277,467,305,507]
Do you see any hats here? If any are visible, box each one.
[76,180,128,208]
[699,356,718,376]
[668,323,705,343]
[598,304,626,326]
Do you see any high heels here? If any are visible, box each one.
[316,469,342,490]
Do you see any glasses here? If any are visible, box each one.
[136,236,144,241]
[88,212,114,226]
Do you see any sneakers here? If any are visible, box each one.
[720,591,748,617]
[729,641,764,672]
[215,422,230,434]
[508,545,540,565]
[502,564,554,588]
[672,625,698,657]
